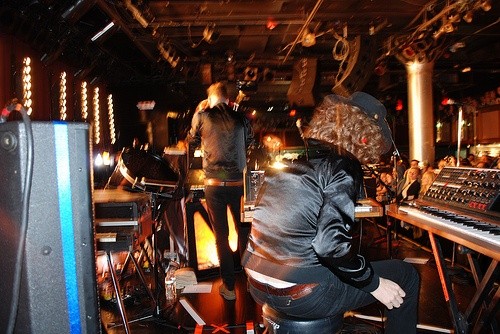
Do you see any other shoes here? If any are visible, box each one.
[218,283,236,300]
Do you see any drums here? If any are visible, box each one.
[104,147,180,229]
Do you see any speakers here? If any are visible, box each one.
[0,120,102,334]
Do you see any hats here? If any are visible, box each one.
[325,88,391,154]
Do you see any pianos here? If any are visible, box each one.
[238,168,384,223]
[386,166,500,261]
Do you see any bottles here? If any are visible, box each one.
[165,270,176,300]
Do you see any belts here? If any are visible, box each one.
[203,178,244,185]
[247,271,317,296]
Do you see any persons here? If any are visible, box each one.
[238,91,420,334]
[187,82,255,299]
[370,151,500,242]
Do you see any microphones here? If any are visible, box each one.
[296,119,307,141]
[447,99,464,106]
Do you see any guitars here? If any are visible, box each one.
[295,118,309,161]
